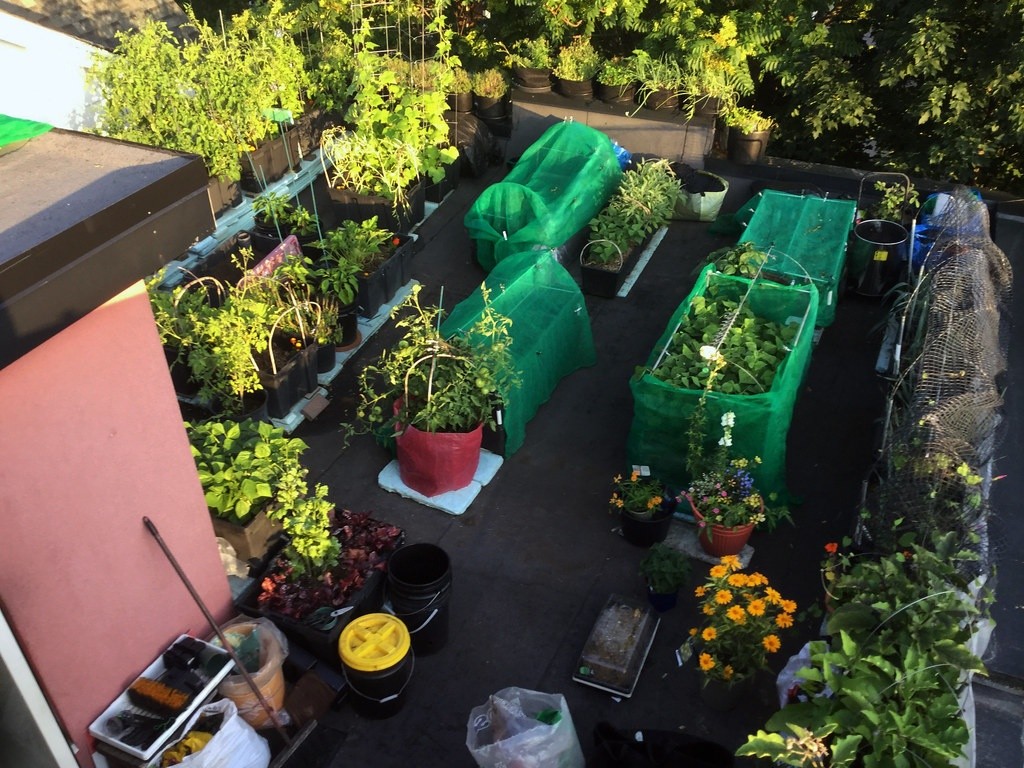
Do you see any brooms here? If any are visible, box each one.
[142,516,319,768]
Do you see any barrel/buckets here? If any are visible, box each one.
[337,612,415,719]
[848,219,908,296]
[383,543,453,656]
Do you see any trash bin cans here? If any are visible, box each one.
[209,625,284,729]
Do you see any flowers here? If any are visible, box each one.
[680,407,771,528]
[675,552,799,679]
[607,467,667,517]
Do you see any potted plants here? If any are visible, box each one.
[97,1,1024,768]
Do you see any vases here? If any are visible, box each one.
[693,667,751,710]
[617,491,676,546]
[687,492,762,555]
